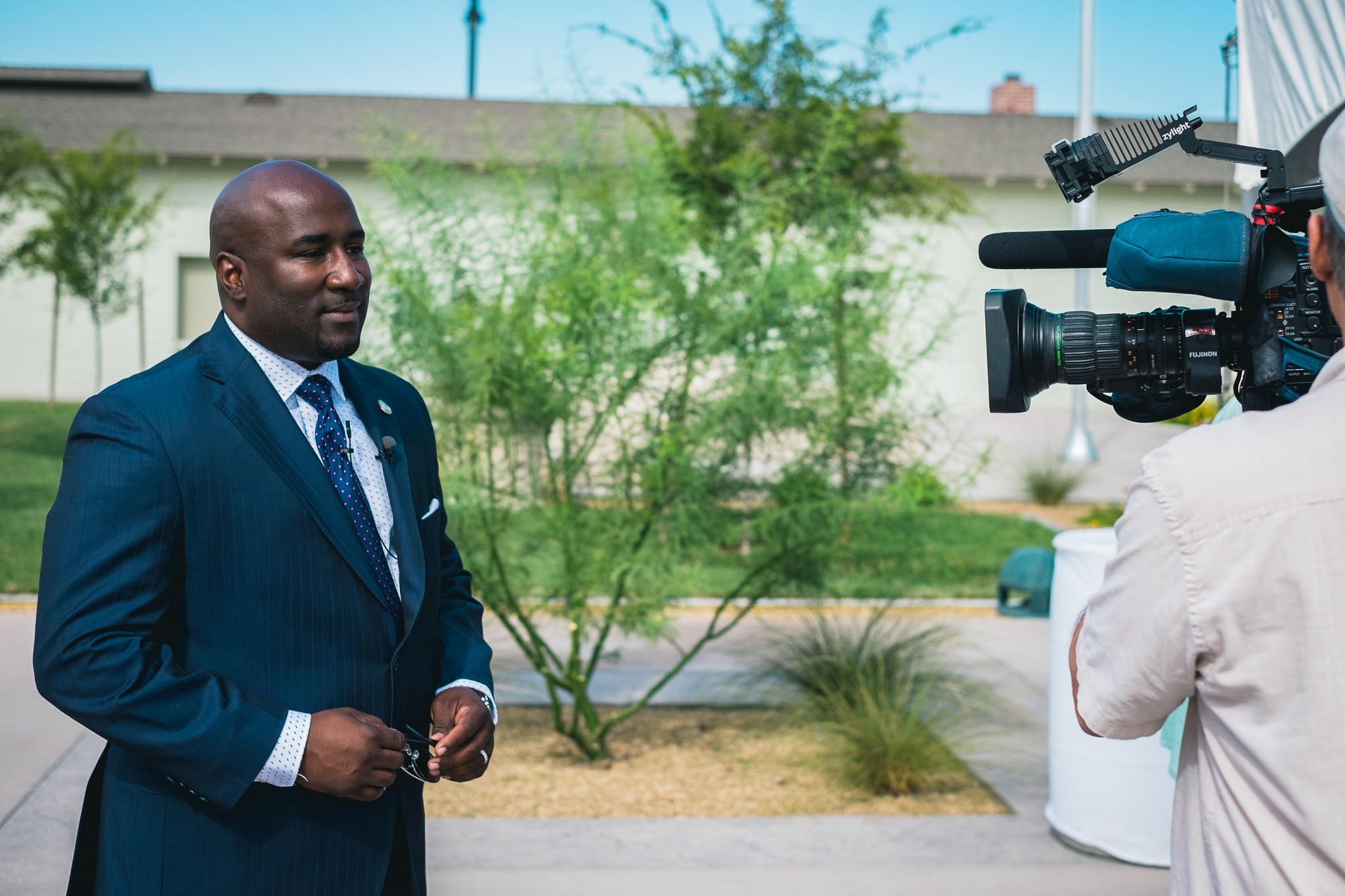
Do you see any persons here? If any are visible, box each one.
[32,157,501,896]
[1066,107,1345,896]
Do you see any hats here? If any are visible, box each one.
[1318,107,1345,245]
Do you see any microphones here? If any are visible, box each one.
[978,229,1116,270]
[346,420,352,439]
[383,436,397,457]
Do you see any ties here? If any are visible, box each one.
[295,372,405,648]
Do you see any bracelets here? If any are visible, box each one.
[467,688,495,722]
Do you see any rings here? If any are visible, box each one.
[480,749,489,764]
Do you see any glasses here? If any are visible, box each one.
[398,721,456,784]
